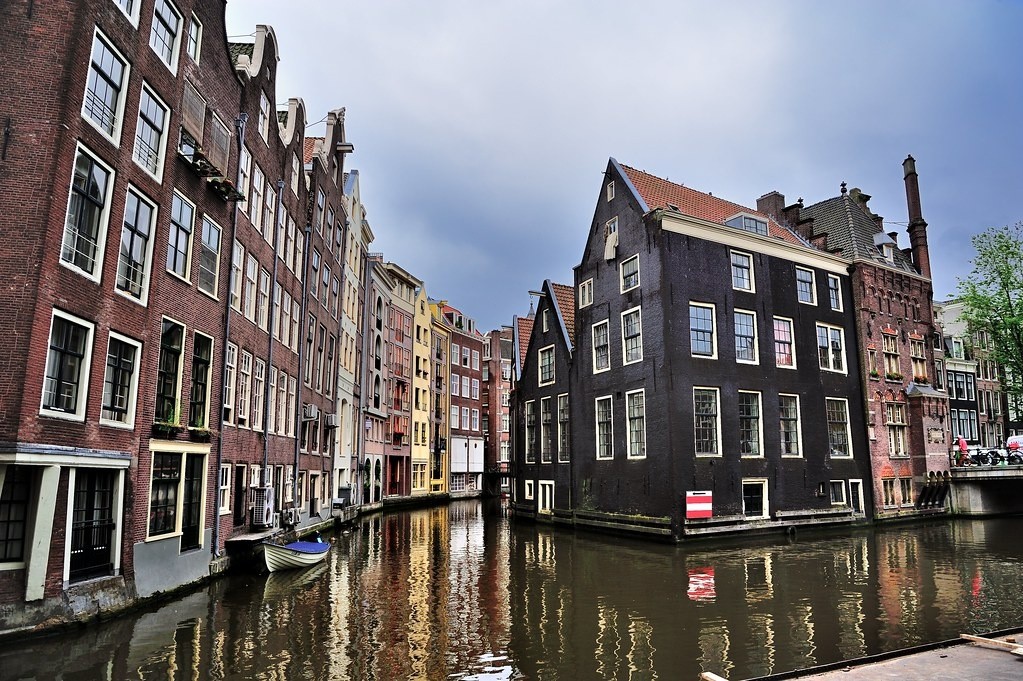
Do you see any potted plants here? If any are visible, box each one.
[223,175,232,184]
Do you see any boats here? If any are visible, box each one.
[261,559,330,605]
[260,528,332,572]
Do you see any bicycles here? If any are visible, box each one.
[954,443,1023,466]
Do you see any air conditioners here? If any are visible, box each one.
[328,414,339,426]
[253,486,274,523]
[282,508,301,524]
[306,404,318,417]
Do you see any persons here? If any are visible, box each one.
[954,435,971,467]
